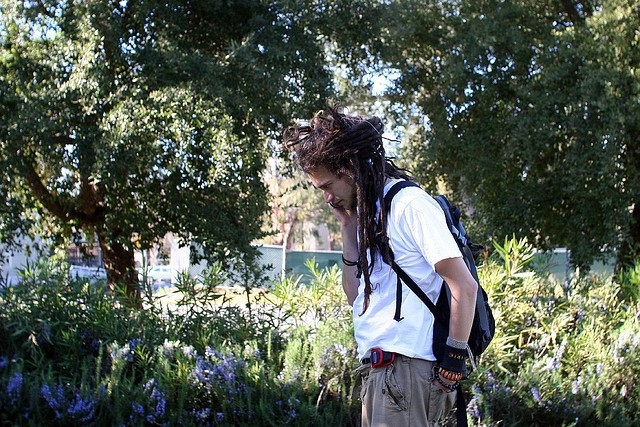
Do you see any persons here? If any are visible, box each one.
[286,105,481,427]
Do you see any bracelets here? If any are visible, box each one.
[436,375,461,390]
[438,366,464,381]
[339,251,362,268]
[439,336,467,372]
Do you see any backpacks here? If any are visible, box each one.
[376,179,495,362]
